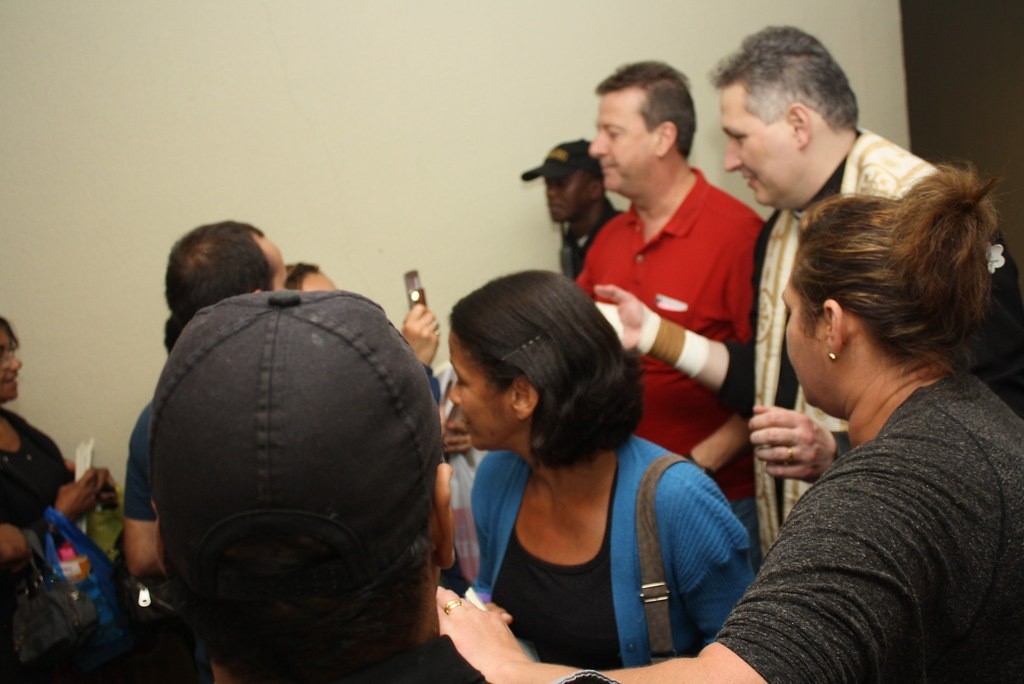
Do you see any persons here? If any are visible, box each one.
[434,166,1024,684]
[0,316,121,684]
[590,22,1024,558]
[570,58,761,556]
[146,289,618,684]
[123,219,492,598]
[443,268,757,671]
[519,138,623,281]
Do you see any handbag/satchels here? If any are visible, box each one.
[41,507,122,643]
[12,528,95,671]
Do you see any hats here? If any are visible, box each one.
[147,289,441,593]
[524,139,603,180]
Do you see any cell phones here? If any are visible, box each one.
[402,271,426,310]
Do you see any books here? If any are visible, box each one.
[72,437,96,535]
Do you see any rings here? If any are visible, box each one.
[444,599,463,614]
[787,446,793,460]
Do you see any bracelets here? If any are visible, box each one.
[685,452,716,479]
[831,432,853,460]
